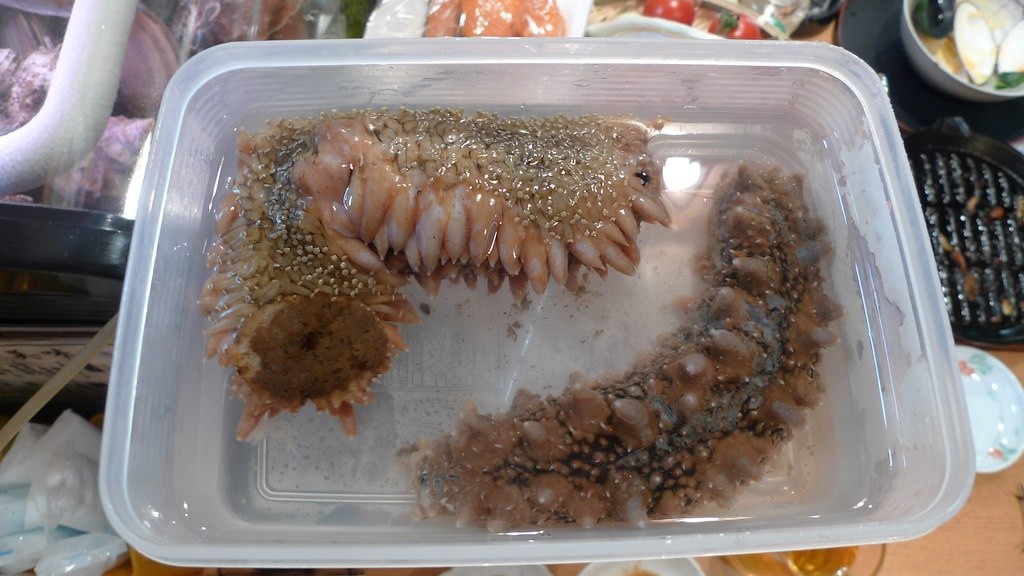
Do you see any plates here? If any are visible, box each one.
[904,346,1023,475]
[581,559,707,576]
[442,565,553,576]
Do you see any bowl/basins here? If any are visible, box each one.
[900,0,1024,102]
[95,39,974,571]
